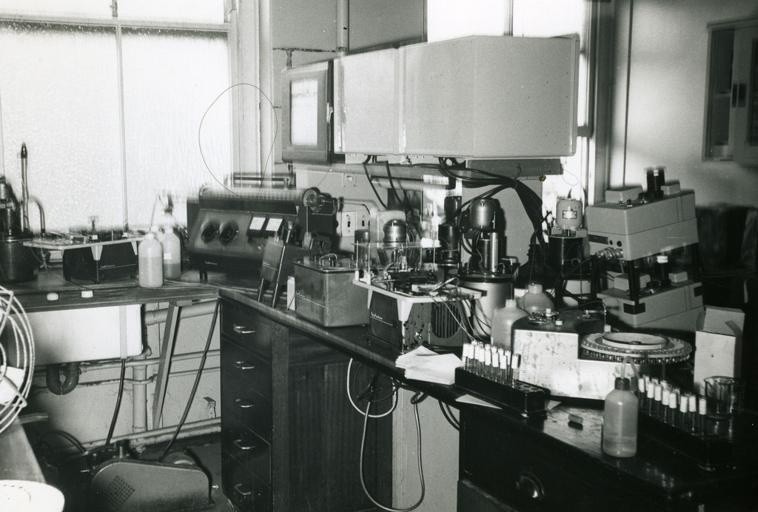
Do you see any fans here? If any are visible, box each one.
[0,285,35,433]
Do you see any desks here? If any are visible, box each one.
[8,280,221,429]
[218,287,758,512]
